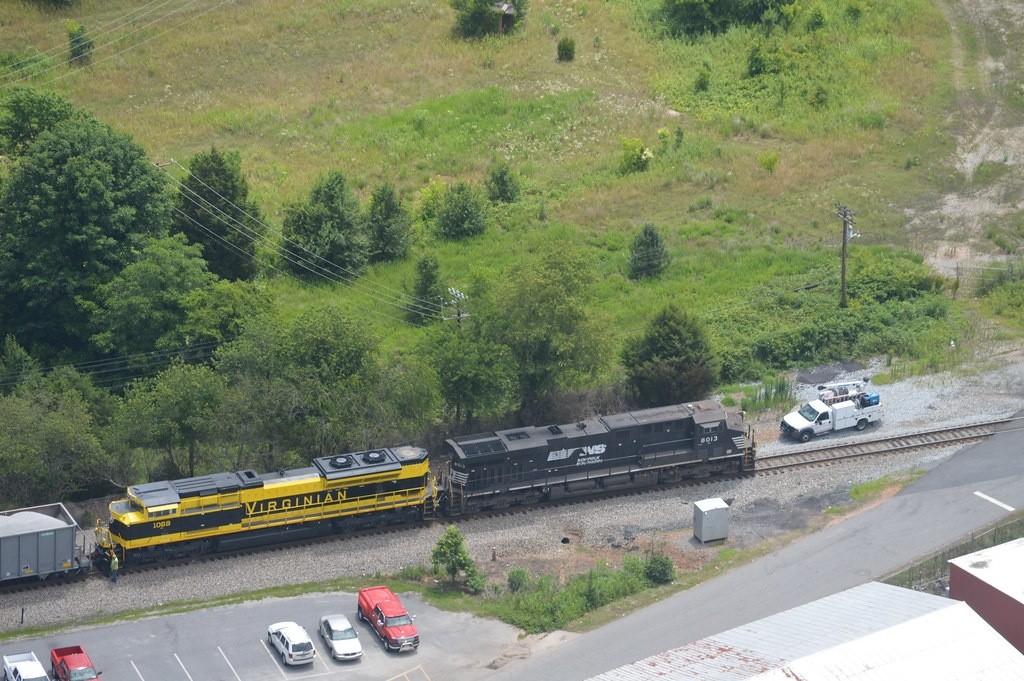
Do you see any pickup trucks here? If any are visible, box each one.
[50,644,102,681]
[2,651,50,681]
[357,585,420,653]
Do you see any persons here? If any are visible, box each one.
[110,555,119,583]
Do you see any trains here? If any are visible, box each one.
[0,395,761,593]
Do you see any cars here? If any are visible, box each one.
[319,613,364,661]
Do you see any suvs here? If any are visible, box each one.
[268,621,318,666]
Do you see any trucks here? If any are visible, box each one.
[779,399,882,444]
